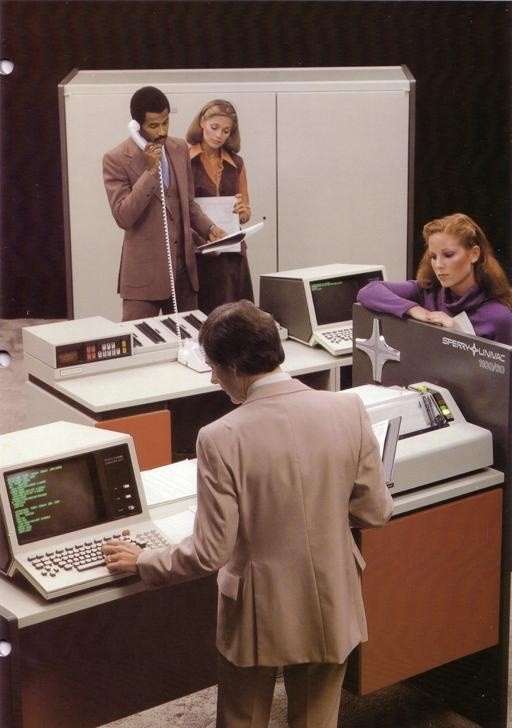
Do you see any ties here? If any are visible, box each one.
[160,153,170,189]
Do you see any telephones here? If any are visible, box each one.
[128,120,150,151]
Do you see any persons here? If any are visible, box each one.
[101,299,395,728]
[102,86,230,323]
[356,212,512,345]
[186,98,254,316]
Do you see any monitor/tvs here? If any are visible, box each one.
[310,271,384,326]
[5,456,106,543]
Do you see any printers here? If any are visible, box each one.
[335,380,505,519]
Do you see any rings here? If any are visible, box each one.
[106,557,112,562]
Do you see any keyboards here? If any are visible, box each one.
[16,522,176,594]
[322,326,353,356]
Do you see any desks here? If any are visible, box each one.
[0,310,512,728]
